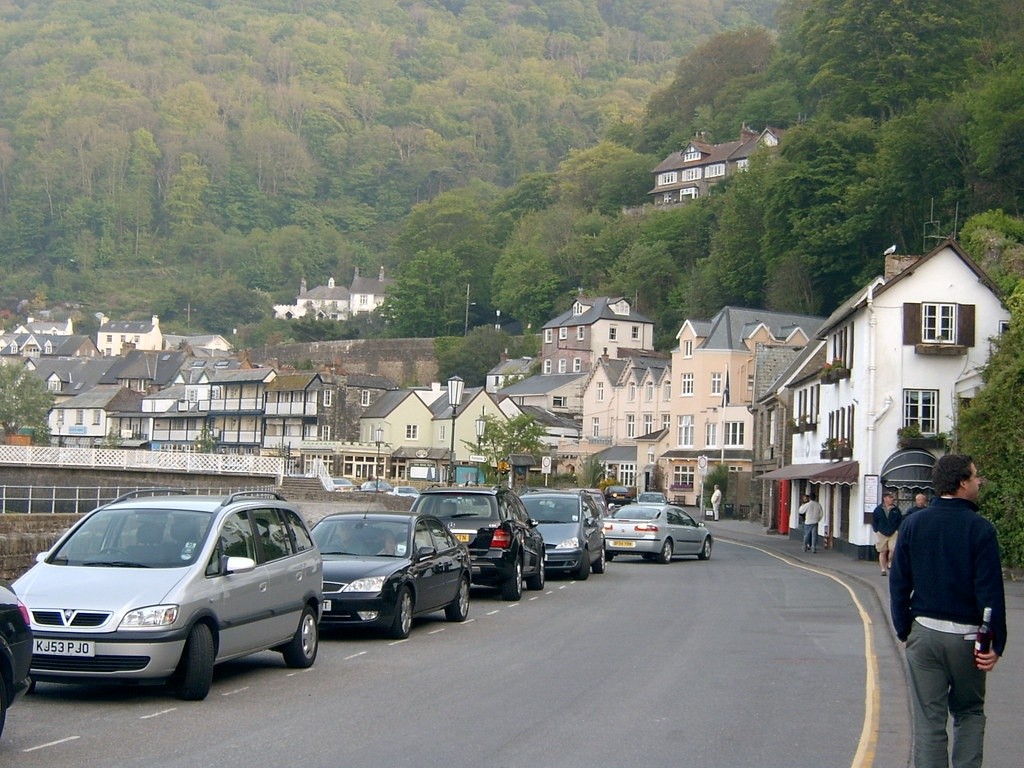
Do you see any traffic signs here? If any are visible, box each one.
[469,455,488,463]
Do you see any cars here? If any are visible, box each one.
[386,486,419,498]
[637,492,668,506]
[566,484,614,521]
[602,486,632,507]
[600,504,713,565]
[308,511,473,639]
[352,482,393,492]
[332,478,357,493]
[0,580,35,738]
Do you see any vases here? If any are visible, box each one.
[831,369,850,379]
[821,375,839,385]
[834,448,851,458]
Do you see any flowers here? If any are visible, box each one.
[830,357,844,371]
[817,362,833,379]
[832,436,849,448]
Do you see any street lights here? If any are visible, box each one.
[446,376,465,487]
[375,428,384,493]
[474,418,486,487]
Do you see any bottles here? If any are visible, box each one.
[974,607,992,668]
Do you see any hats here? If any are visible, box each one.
[883,491,893,496]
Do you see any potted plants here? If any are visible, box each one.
[797,412,817,432]
[786,417,804,435]
[897,421,947,450]
[820,437,842,459]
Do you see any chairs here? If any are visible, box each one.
[439,501,457,514]
[125,523,169,562]
[161,519,199,560]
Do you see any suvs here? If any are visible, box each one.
[410,486,547,602]
[7,486,324,702]
[516,487,606,583]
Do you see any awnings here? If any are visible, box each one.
[881,447,938,490]
[755,461,859,486]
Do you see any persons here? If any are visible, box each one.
[710,484,722,521]
[889,453,1007,768]
[379,532,404,556]
[906,494,928,517]
[871,491,902,577]
[328,523,369,555]
[798,492,823,554]
